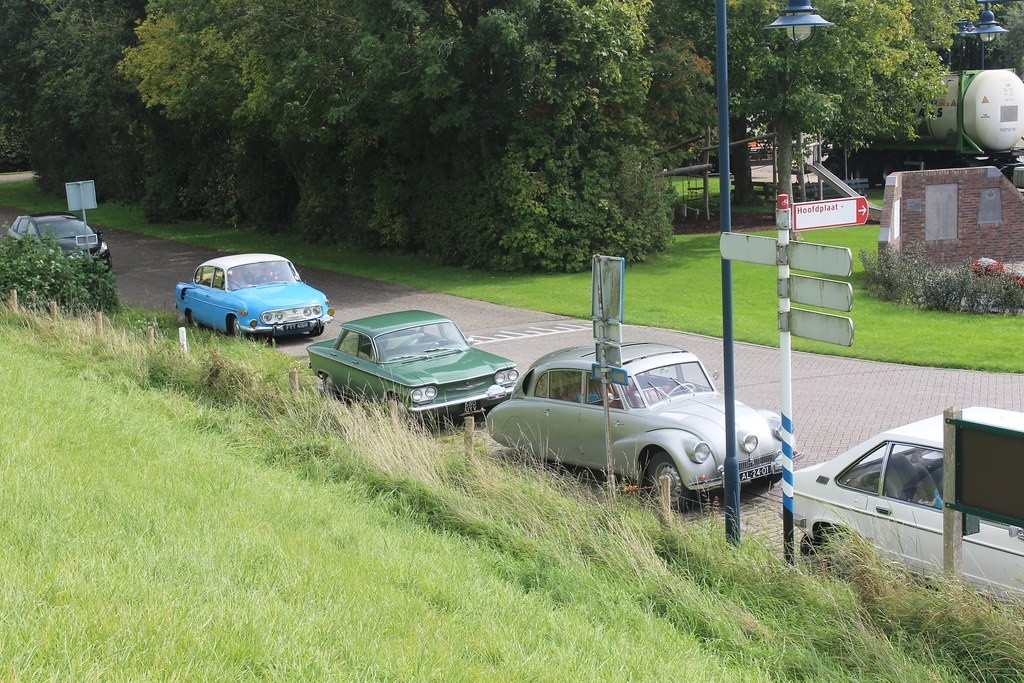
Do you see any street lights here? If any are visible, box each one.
[949,0,1008,169]
[711,1,835,546]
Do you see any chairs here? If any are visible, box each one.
[361,332,426,357]
[379,342,438,363]
[567,378,638,407]
[627,384,672,406]
[873,454,938,506]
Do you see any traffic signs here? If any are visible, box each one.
[719,192,870,566]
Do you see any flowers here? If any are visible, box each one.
[971,257,1004,276]
[1007,267,1024,288]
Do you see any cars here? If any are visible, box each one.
[784,413,1023,618]
[484,341,801,516]
[308,309,521,434]
[8,213,114,274]
[174,252,332,344]
